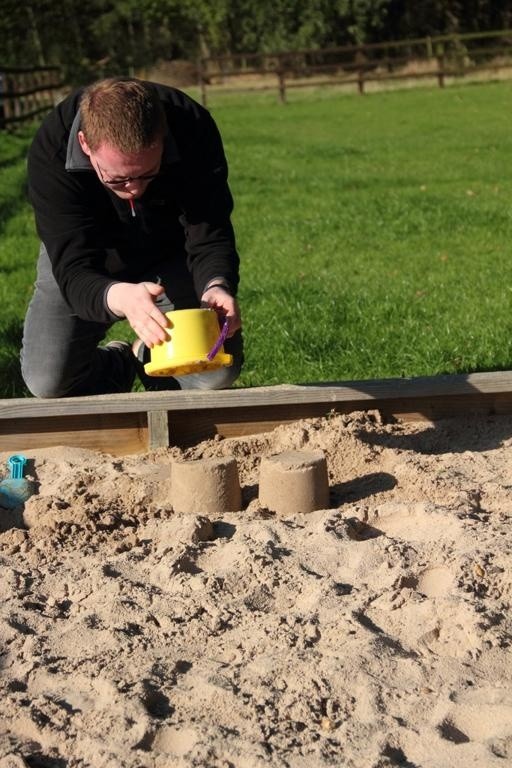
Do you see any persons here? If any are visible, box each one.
[16,74,244,401]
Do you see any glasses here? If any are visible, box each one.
[97,161,159,184]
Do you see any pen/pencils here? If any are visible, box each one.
[129,200,137,218]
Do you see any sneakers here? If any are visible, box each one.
[106,338,179,393]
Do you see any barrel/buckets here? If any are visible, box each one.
[144,308,234,377]
